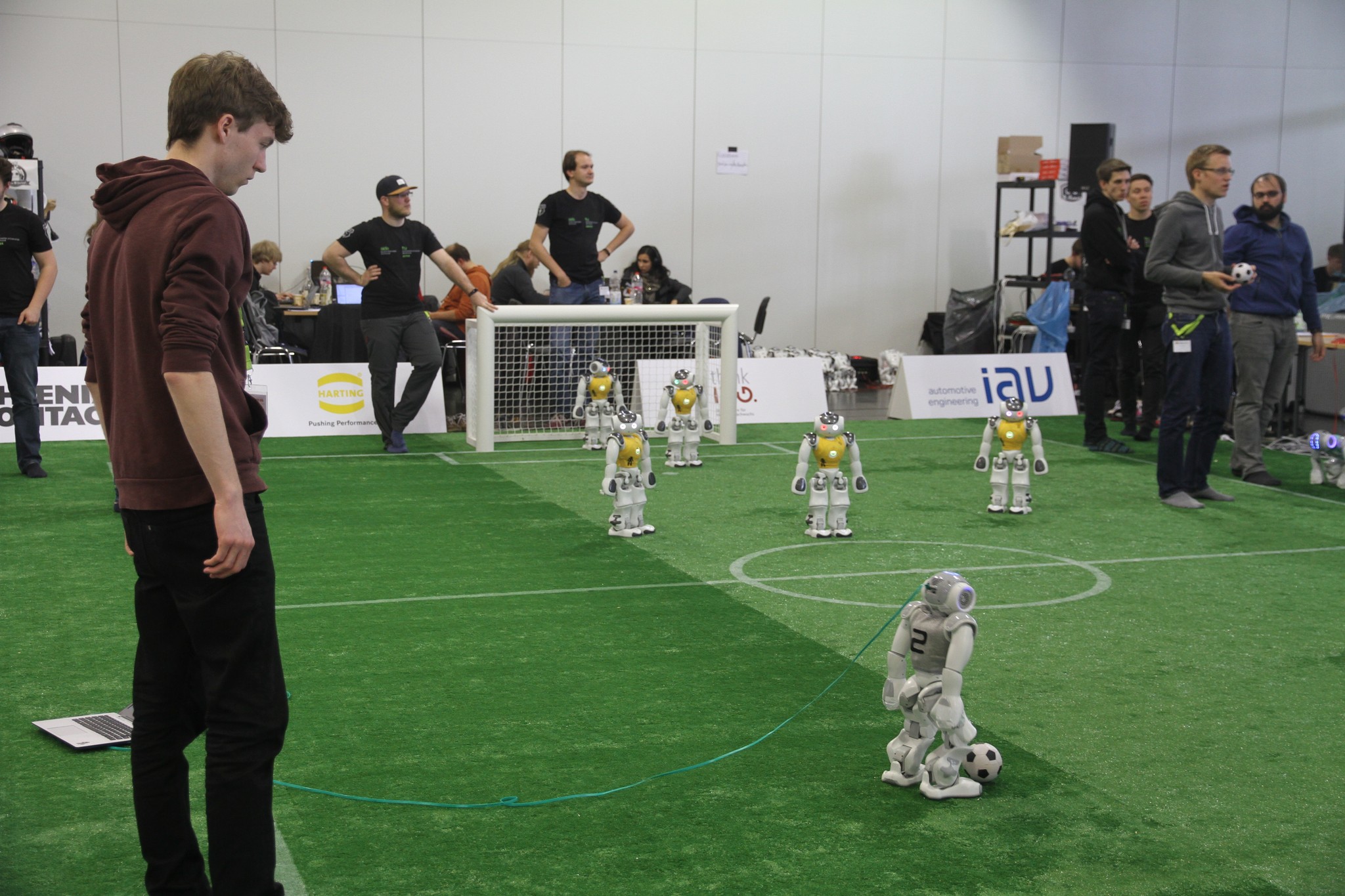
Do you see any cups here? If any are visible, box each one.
[292,294,302,306]
[311,293,320,305]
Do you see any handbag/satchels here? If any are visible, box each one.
[643,282,661,302]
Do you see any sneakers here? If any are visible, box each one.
[504,416,526,428]
[573,418,586,428]
[446,413,466,432]
[545,414,570,429]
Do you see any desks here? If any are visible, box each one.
[1276,332,1345,441]
[280,308,322,317]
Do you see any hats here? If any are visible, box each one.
[376,175,417,200]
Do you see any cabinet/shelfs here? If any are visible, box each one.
[2,156,52,366]
[992,178,1090,401]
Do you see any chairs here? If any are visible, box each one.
[241,291,308,363]
[439,296,495,398]
[659,292,773,359]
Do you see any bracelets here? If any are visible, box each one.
[604,248,610,256]
[468,287,478,297]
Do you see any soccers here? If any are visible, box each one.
[962,742,1003,782]
[1231,263,1252,283]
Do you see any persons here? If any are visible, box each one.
[572,360,997,800]
[426,243,492,433]
[30,191,56,356]
[621,246,694,355]
[322,175,498,454]
[1308,430,1345,489]
[250,240,312,364]
[1143,145,1257,508]
[490,239,550,429]
[973,398,1049,513]
[1047,145,1160,454]
[77,45,294,896]
[0,156,59,478]
[1221,174,1326,487]
[528,149,636,426]
[1313,243,1345,292]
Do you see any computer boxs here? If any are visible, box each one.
[311,260,337,300]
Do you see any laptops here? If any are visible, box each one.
[280,285,319,310]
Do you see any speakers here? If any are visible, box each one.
[1068,123,1115,192]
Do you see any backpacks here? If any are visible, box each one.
[243,289,279,350]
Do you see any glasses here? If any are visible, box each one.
[1198,166,1236,176]
[396,192,413,199]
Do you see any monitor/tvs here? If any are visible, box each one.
[336,282,364,304]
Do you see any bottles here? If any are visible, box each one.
[608,271,621,305]
[632,272,643,305]
[320,267,332,306]
[303,269,313,298]
